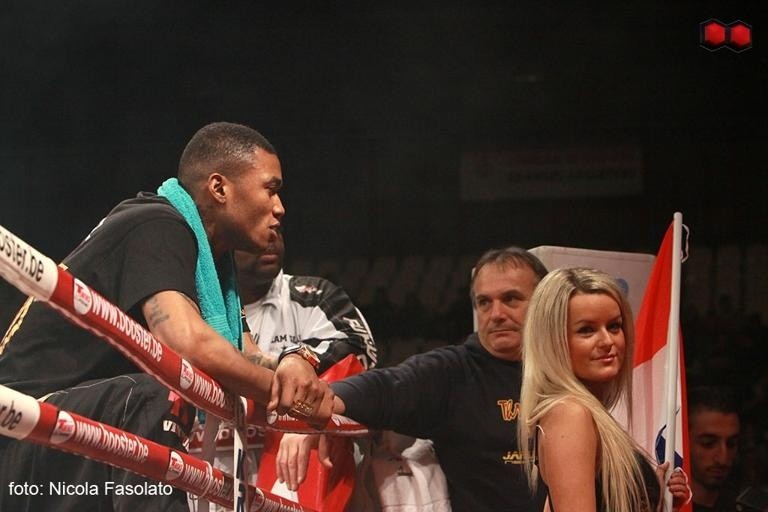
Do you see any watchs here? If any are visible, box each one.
[277,343,322,375]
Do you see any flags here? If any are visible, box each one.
[604,207,695,510]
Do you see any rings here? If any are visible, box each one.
[289,398,314,423]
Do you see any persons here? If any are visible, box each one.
[516,266,694,511]
[685,386,768,511]
[180,214,382,512]
[268,247,550,512]
[1,119,299,511]
